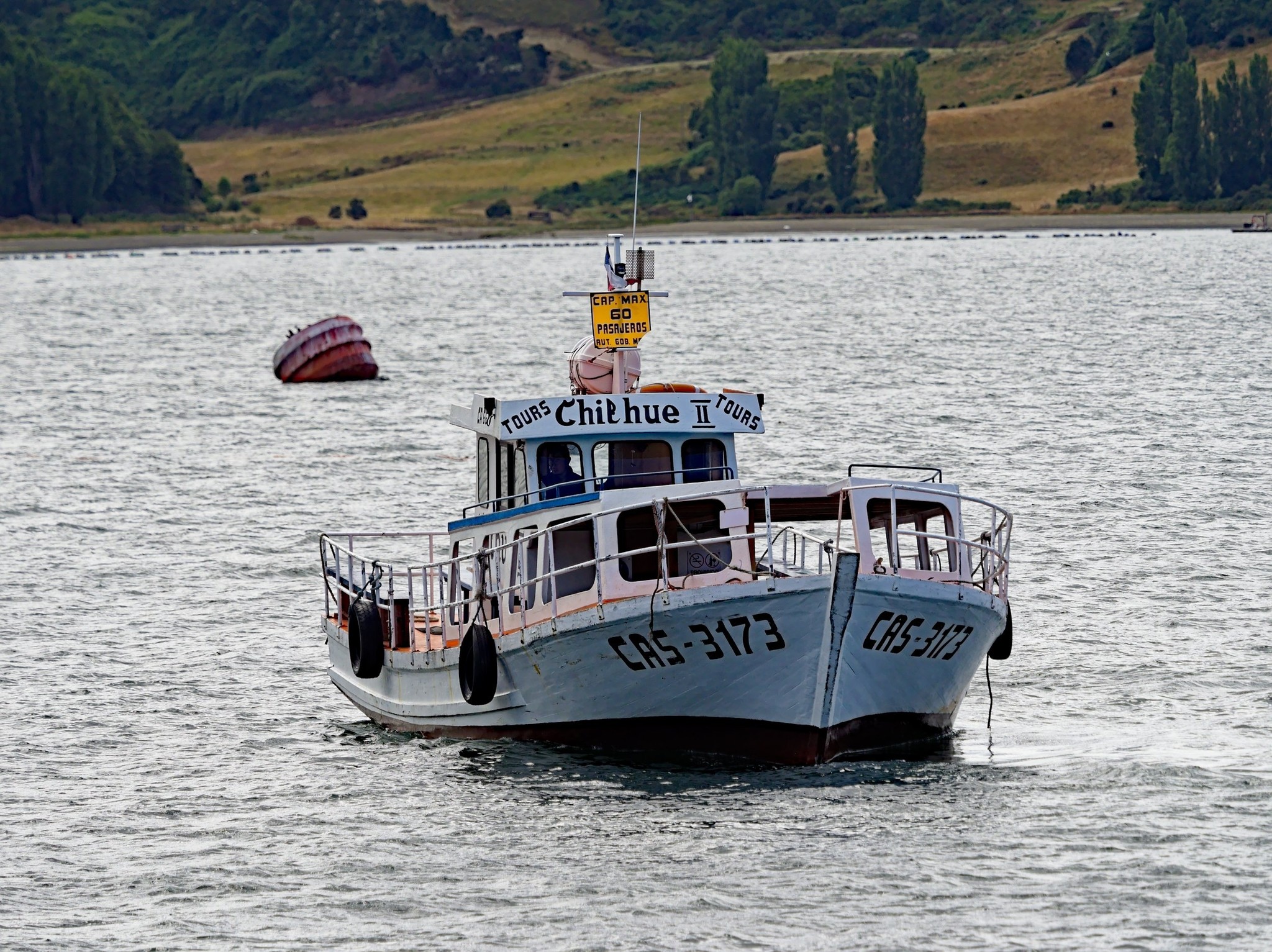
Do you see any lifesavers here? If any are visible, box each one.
[987,593,1013,661]
[631,381,708,394]
[348,600,385,680]
[457,623,500,706]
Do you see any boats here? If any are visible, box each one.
[316,112,1014,767]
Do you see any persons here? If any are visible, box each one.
[595,440,650,491]
[540,445,591,530]
